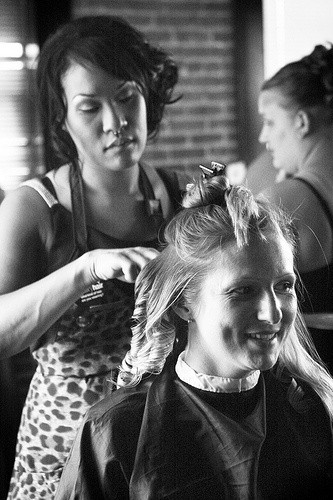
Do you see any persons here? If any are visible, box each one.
[252,42,333,376]
[55,178,333,500]
[0,15,200,500]
